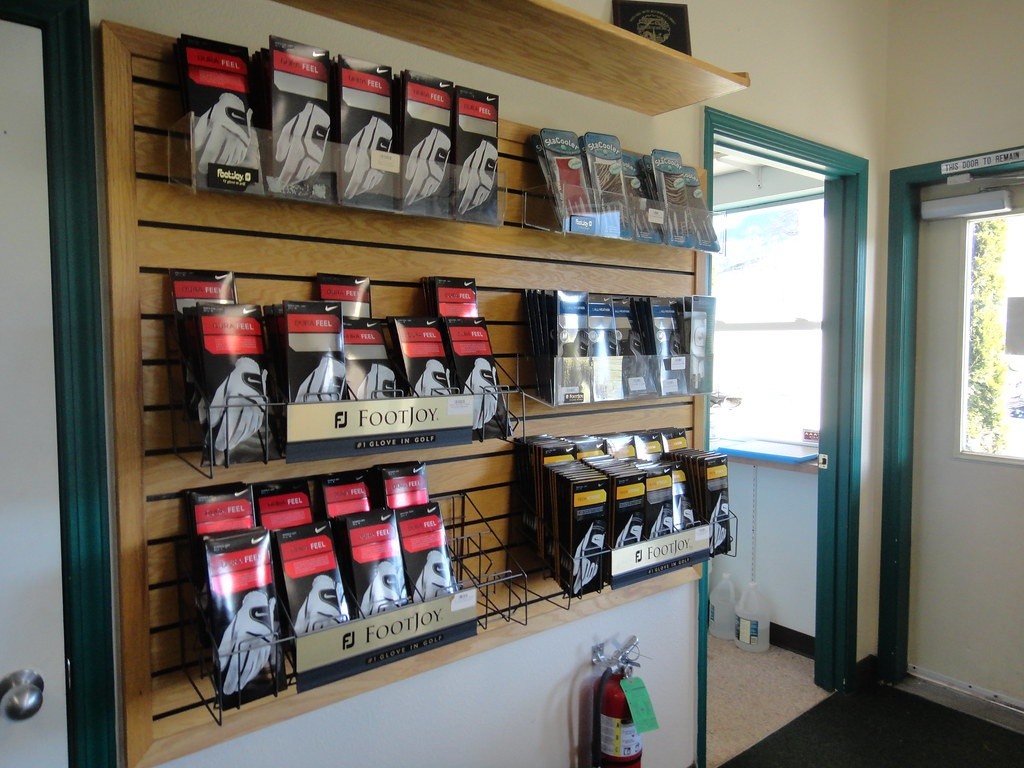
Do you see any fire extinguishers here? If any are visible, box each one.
[592,636,659,768]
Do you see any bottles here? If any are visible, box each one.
[710,573,736,641]
[735,583,771,652]
